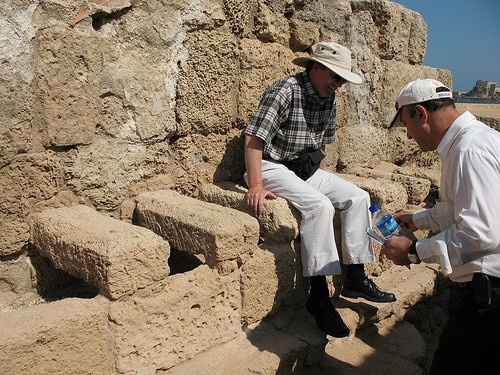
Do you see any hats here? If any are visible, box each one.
[388,79,453,130]
[292,42,362,85]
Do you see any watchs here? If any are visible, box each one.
[407,240,421,264]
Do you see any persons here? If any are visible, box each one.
[244,40,397,337]
[379,79,500,375]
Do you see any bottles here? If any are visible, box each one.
[369,204,401,239]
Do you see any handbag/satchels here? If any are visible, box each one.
[285,149,325,180]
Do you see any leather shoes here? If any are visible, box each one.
[341,277,396,303]
[306,296,350,338]
